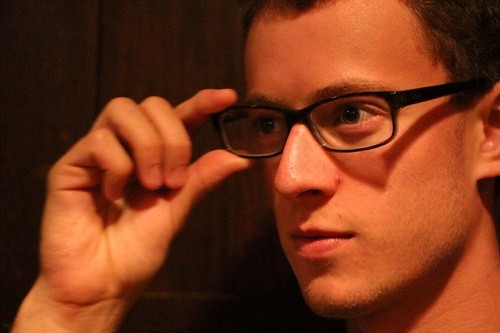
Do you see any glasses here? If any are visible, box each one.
[208,77,492,158]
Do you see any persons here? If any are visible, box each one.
[13,1,500,333]
